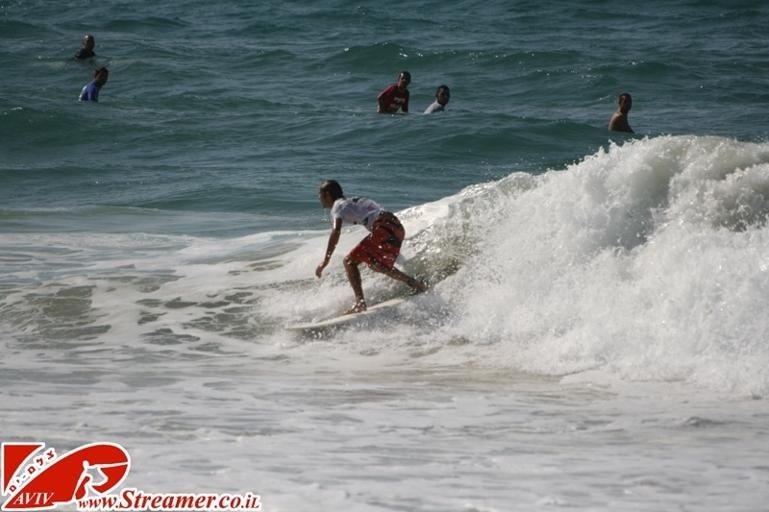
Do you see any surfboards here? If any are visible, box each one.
[283,272,458,330]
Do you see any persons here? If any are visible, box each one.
[75,67,109,103]
[607,93,635,134]
[376,71,413,114]
[72,34,96,62]
[311,179,431,316]
[421,84,451,115]
